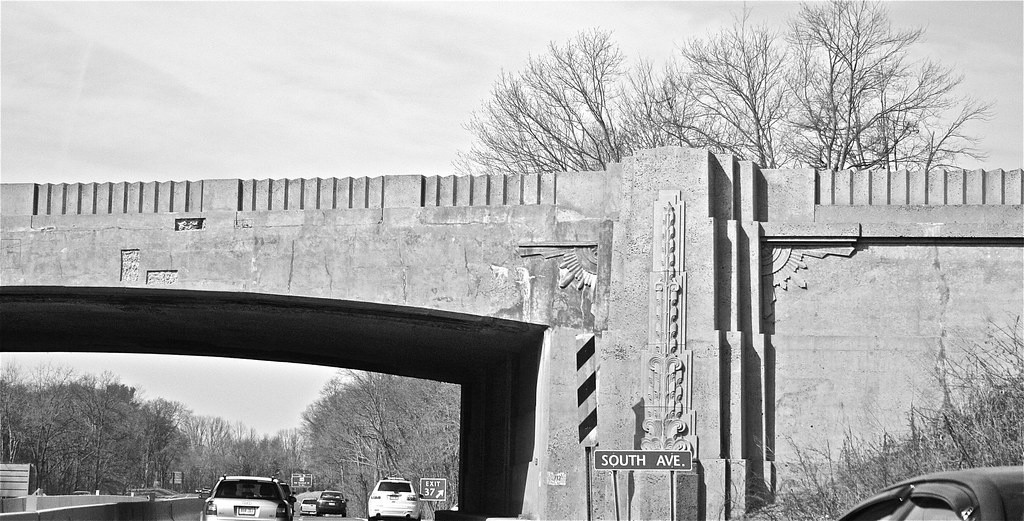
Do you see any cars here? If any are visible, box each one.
[300,498,319,516]
[833,464,1023,521]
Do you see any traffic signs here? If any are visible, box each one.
[419,478,447,502]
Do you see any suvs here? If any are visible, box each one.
[367,477,424,521]
[316,490,349,517]
[199,473,297,521]
